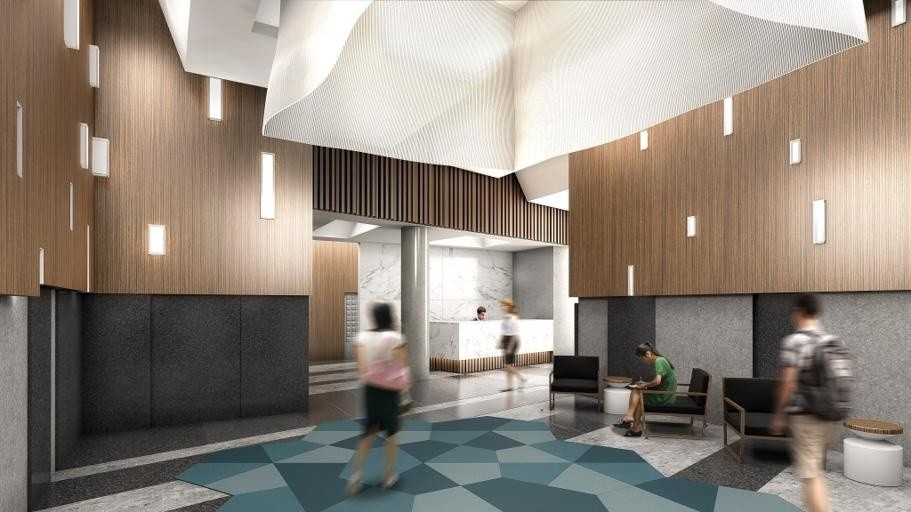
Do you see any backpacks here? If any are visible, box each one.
[800,331,858,419]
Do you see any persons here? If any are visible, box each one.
[770,293,853,511]
[473,307,486,320]
[344,303,410,492]
[612,341,677,437]
[495,298,527,393]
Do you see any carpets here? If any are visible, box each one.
[175,416,802,512]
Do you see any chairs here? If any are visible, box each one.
[721,378,779,464]
[636,368,712,439]
[549,356,601,413]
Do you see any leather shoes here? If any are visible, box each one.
[613,418,631,430]
[624,428,643,437]
